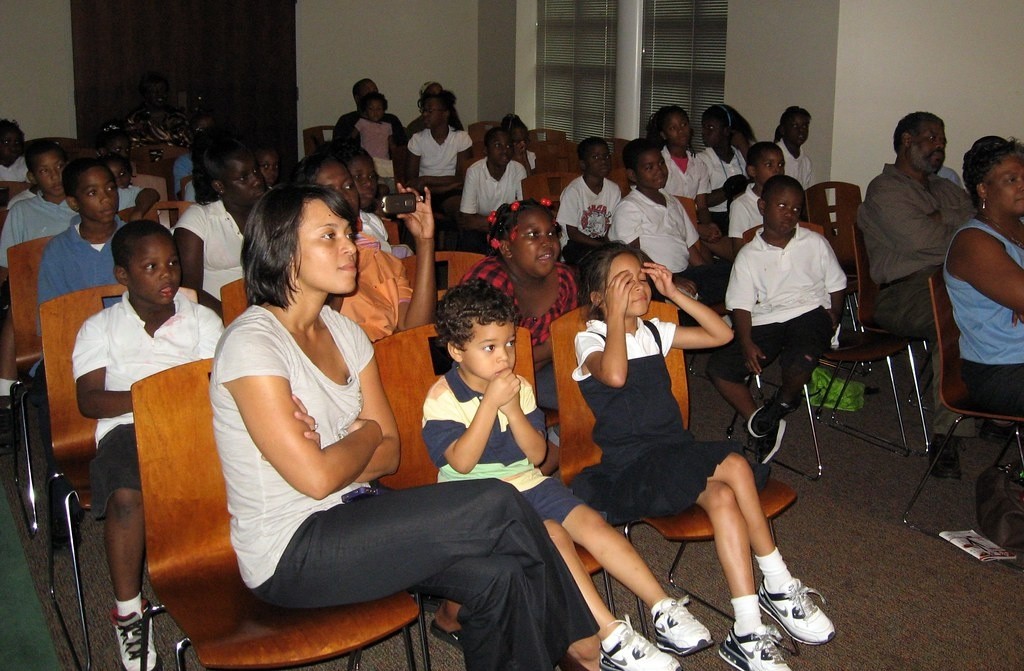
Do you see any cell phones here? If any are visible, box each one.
[381,193,417,214]
[342,487,391,504]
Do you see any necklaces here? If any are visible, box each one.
[978,211,1024,251]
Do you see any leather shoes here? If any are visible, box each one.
[929,437,959,478]
[980,418,1024,442]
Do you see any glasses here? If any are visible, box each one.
[419,107,446,114]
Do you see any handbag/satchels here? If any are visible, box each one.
[976,462,1024,547]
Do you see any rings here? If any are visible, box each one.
[418,198,422,202]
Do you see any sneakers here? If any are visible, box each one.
[653,594,714,654]
[758,577,836,645]
[748,386,803,438]
[110,596,160,671]
[718,621,791,671]
[599,613,680,671]
[759,418,786,463]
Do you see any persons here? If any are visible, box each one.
[571,241,836,671]
[423,279,715,671]
[936,165,961,186]
[72,221,226,671]
[421,196,579,650]
[942,132,1024,420]
[857,111,1024,483]
[209,182,607,671]
[1,71,848,554]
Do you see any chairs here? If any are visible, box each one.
[0,122,1024,671]
